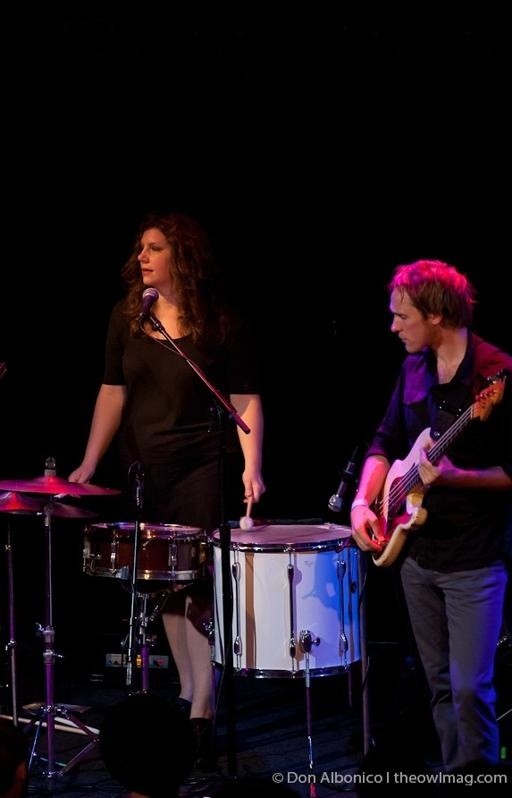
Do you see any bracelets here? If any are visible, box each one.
[350,501,371,511]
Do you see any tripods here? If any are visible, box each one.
[26,501,99,782]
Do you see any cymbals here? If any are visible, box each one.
[0,492,41,511]
[0,476,124,496]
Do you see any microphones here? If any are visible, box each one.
[327,460,356,513]
[141,288,159,318]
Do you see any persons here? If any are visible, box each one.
[66,208,265,729]
[347,258,509,774]
[0,688,512,798]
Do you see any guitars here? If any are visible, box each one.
[370,370,505,569]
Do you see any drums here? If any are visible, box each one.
[207,522,368,681]
[81,522,206,581]
